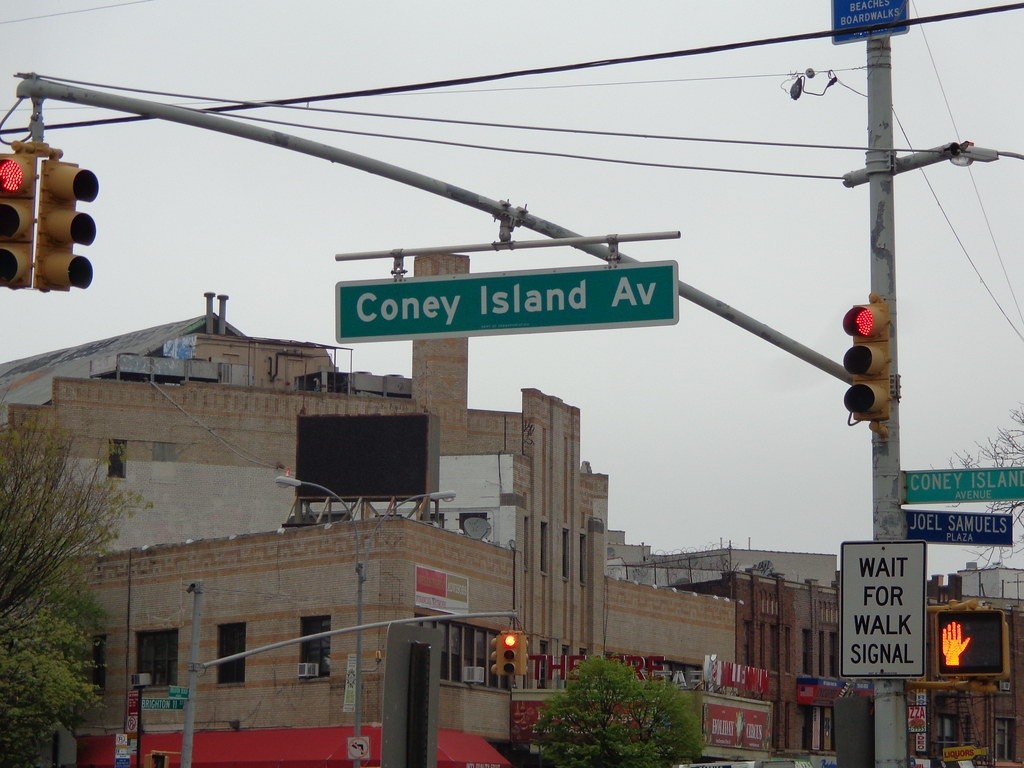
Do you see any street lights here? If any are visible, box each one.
[274,474,457,768]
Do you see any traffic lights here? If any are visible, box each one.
[930,608,1011,680]
[32,159,99,291]
[0,154,39,290]
[495,633,527,676]
[842,303,892,420]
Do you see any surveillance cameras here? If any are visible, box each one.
[191,580,202,588]
[186,585,193,593]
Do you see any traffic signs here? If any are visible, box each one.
[128,691,139,716]
